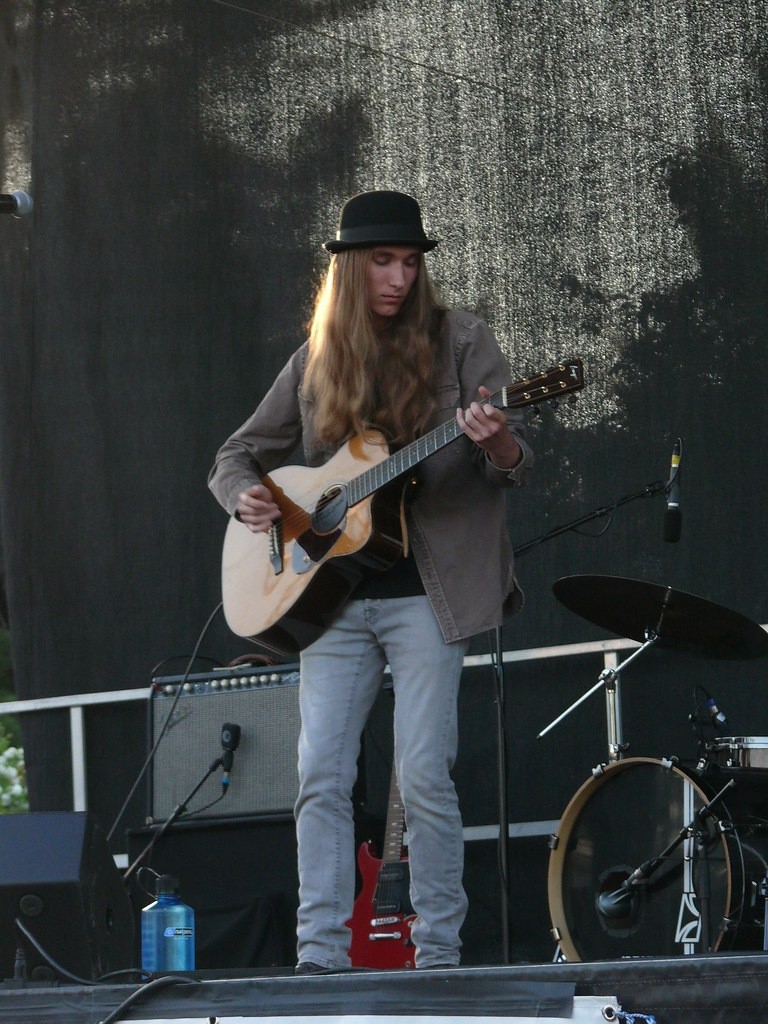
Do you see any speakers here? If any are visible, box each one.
[0,810,135,985]
[146,663,303,826]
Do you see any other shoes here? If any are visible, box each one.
[295,962,326,973]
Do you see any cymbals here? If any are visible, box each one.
[550,572,768,661]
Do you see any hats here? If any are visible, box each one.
[325,190,438,253]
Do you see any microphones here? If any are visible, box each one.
[221,724,241,793]
[595,860,655,919]
[0,190,35,218]
[662,442,682,543]
[704,690,726,729]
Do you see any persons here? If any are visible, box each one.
[207,191,533,974]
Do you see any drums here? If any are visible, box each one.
[546,755,768,963]
[705,735,768,775]
[345,755,418,969]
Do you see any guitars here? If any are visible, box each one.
[221,355,585,658]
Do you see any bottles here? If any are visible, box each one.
[137,867,195,982]
